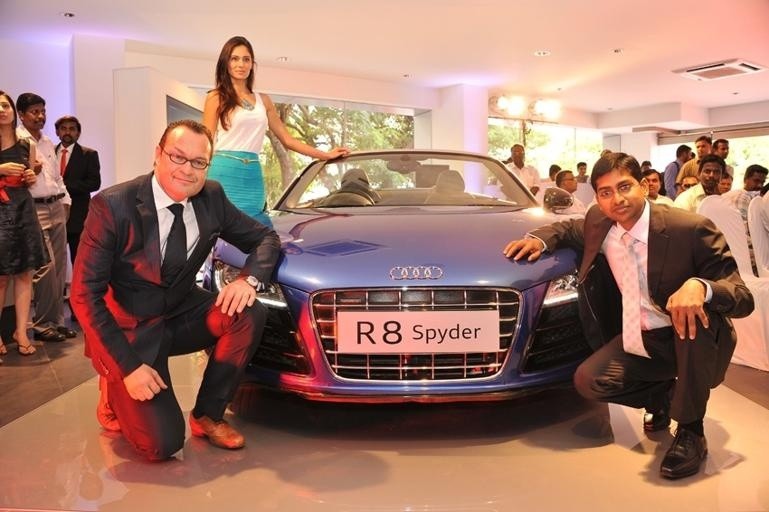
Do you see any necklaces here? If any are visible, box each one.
[236,91,254,110]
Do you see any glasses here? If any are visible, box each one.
[161,147,209,169]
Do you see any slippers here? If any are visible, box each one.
[13,340,32,356]
[57,327,77,337]
[34,328,66,342]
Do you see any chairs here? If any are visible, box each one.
[698,189,768,374]
[339,168,380,206]
[425,170,474,206]
[536,182,595,210]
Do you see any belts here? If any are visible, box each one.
[34,193,65,204]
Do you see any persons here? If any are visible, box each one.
[501,137,768,272]
[202,36,351,217]
[70,119,282,460]
[54,115,101,322]
[502,153,755,479]
[0,89,51,357]
[16,92,77,341]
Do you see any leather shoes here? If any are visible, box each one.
[189,409,245,448]
[660,429,707,478]
[643,378,678,430]
[98,374,120,432]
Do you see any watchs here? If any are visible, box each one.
[237,274,263,293]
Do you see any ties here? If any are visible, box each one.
[60,149,67,175]
[161,203,187,284]
[622,232,651,358]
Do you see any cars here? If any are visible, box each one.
[202,147,630,419]
